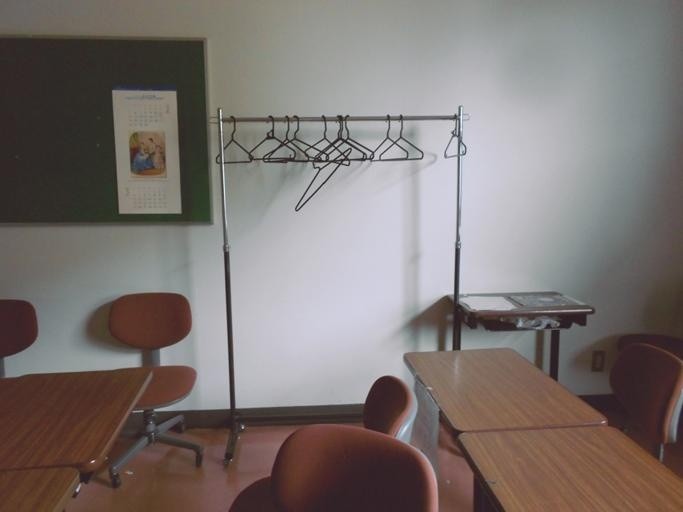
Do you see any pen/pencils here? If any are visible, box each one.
[508,296,524,307]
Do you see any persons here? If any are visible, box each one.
[151,144,164,168]
[131,141,152,170]
[146,138,155,157]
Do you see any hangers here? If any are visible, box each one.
[215,115,467,212]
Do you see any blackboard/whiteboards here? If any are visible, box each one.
[0,35,214,227]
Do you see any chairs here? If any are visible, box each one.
[610,343,683,464]
[0,298,38,378]
[106,291,204,482]
[226,377,439,511]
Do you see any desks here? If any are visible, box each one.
[0,366,150,511]
[448,291,595,385]
